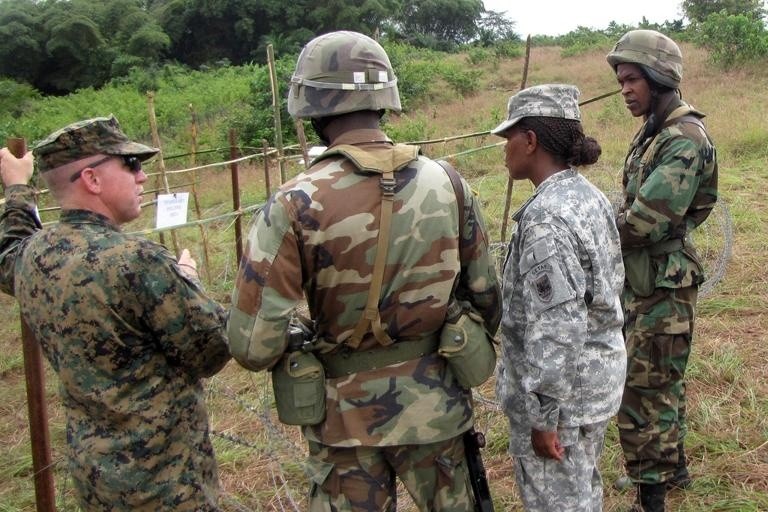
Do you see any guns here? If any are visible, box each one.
[464,427,494,512]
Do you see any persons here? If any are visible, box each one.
[492,82,628,512]
[223,29,502,512]
[0,113,230,512]
[607,29,720,512]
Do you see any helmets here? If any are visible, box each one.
[288,31,402,118]
[606,30,683,88]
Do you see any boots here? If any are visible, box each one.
[614,442,690,492]
[628,484,664,512]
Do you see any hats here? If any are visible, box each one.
[33,114,160,173]
[491,84,580,138]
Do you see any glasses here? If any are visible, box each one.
[70,155,140,182]
